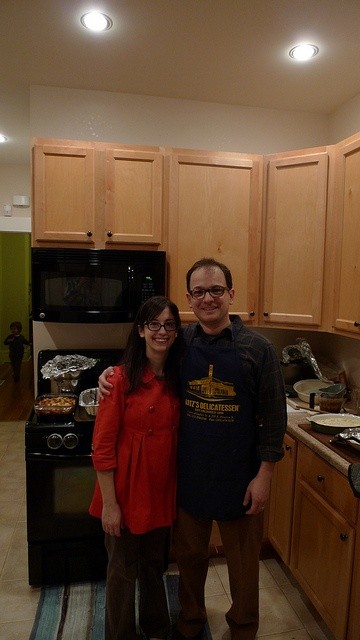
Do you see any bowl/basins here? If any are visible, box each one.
[306,412,360,436]
[293,379,334,405]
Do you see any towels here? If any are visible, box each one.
[347,463,360,498]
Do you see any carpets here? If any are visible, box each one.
[28,575,212,640]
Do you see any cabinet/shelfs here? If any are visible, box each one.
[288,441,360,639]
[260,146,328,333]
[329,132,360,340]
[265,433,296,567]
[29,138,165,248]
[165,148,260,329]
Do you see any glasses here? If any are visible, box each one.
[142,320,179,331]
[188,286,229,298]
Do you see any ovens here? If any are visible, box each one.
[24,424,172,586]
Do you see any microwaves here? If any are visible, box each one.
[30,246,168,325]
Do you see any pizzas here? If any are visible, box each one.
[38,397,75,414]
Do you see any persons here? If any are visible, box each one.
[4,321,32,382]
[90,296,181,639]
[95,258,288,639]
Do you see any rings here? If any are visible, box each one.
[260,507,265,512]
